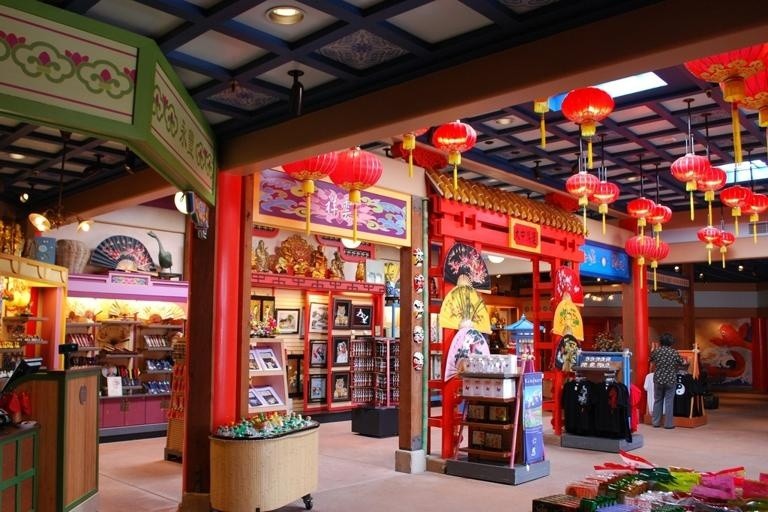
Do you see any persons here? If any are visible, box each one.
[648,331,689,429]
[331,251,346,278]
[255,240,270,273]
[311,244,328,266]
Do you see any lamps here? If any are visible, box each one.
[28,131,97,234]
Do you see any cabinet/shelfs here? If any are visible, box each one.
[446,344,548,483]
[66,319,186,437]
[248,338,289,416]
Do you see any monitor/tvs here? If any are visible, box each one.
[0,357,43,392]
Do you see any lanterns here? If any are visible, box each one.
[392,122,431,178]
[558,85,615,168]
[564,152,768,293]
[328,147,385,242]
[426,120,478,190]
[718,71,768,129]
[684,42,768,165]
[530,96,550,149]
[280,148,340,237]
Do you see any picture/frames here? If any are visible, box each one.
[276,299,373,404]
